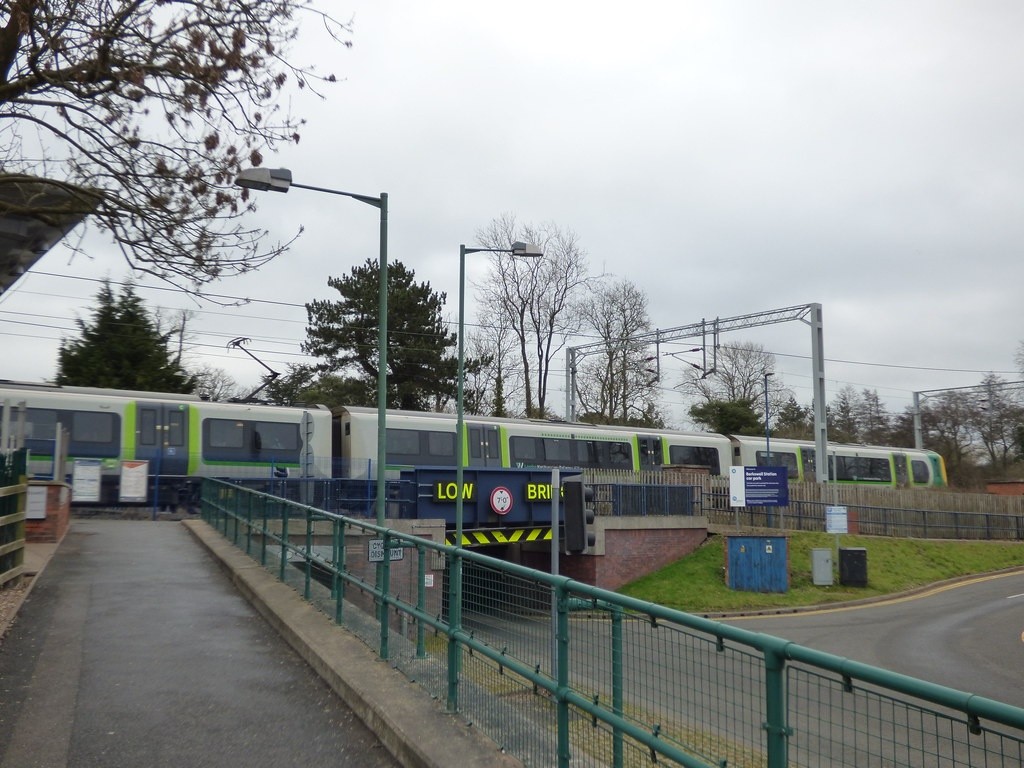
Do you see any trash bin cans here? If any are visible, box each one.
[839,546,868,587]
[812,547,835,586]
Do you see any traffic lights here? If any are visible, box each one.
[565,482,595,557]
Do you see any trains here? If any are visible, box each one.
[0,379,948,508]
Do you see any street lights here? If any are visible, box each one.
[458,241,545,544]
[235,168,389,621]
[765,373,775,467]
